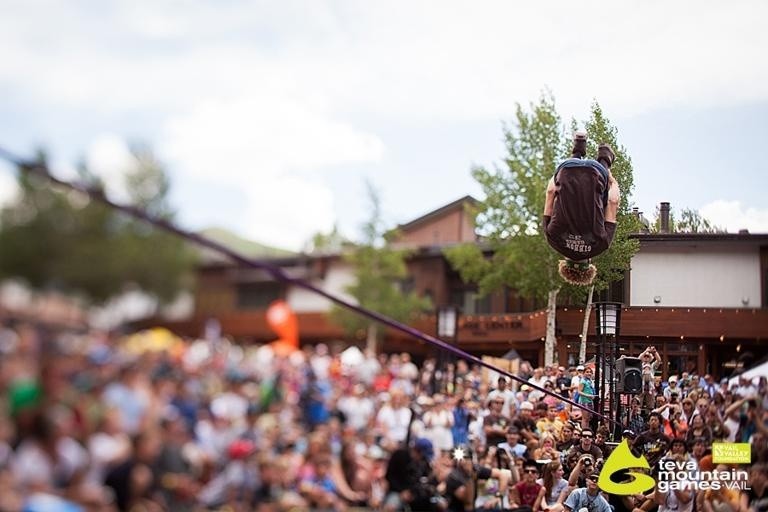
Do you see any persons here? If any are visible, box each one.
[543,132,622,287]
[2,264,768,512]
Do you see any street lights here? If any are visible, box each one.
[587,298,626,453]
[428,298,463,401]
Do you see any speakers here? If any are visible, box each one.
[615,357,643,394]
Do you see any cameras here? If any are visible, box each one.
[668,408,676,426]
[582,456,593,467]
[582,500,597,512]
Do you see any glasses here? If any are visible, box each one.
[589,476,599,481]
[644,355,649,358]
[582,435,593,438]
[524,470,536,473]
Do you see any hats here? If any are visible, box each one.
[622,427,636,439]
[521,384,529,390]
[414,439,433,461]
[576,366,585,371]
[669,376,676,383]
[519,401,534,411]
